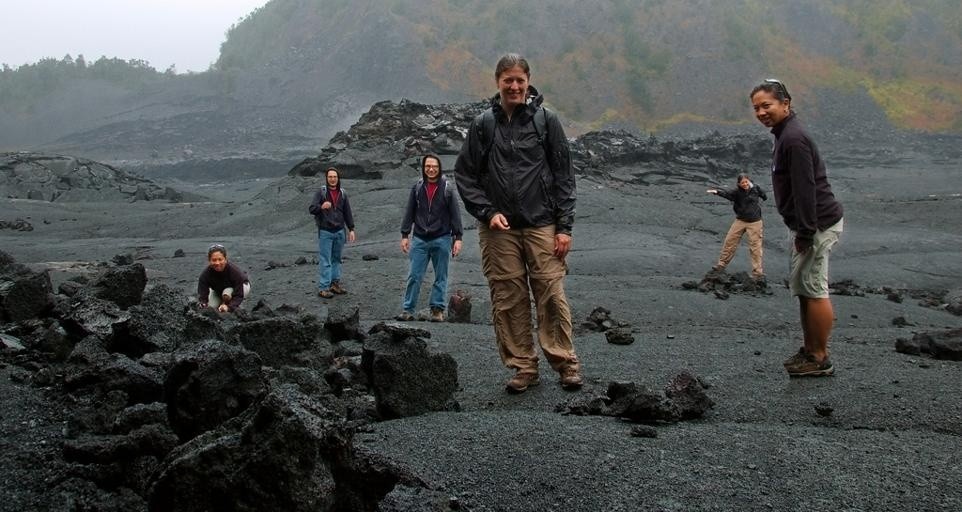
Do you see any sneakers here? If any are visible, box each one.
[561,368,583,389]
[330,282,346,294]
[788,355,834,375]
[507,374,541,391]
[783,347,808,366]
[396,311,416,321]
[429,307,446,322]
[712,265,725,271]
[318,291,334,298]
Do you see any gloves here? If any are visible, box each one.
[794,240,812,253]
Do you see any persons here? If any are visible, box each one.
[454,52,585,392]
[704,174,767,278]
[310,167,356,299]
[749,79,844,378]
[200,245,253,316]
[397,155,464,321]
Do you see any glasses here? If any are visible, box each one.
[764,79,786,97]
[208,245,225,253]
